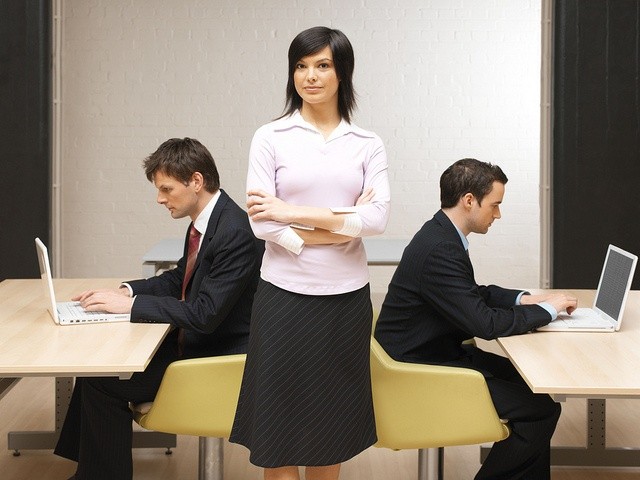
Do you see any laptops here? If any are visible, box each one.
[533,244,638,332]
[33,237,132,326]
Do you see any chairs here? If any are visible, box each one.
[368,310,512,479]
[129,351,248,480]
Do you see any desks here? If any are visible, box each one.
[1,278,177,457]
[141,236,410,277]
[479,283,639,480]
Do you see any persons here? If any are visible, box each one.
[245,24,389,480]
[53,135,266,479]
[373,158,579,479]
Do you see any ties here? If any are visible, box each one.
[178,225,202,356]
